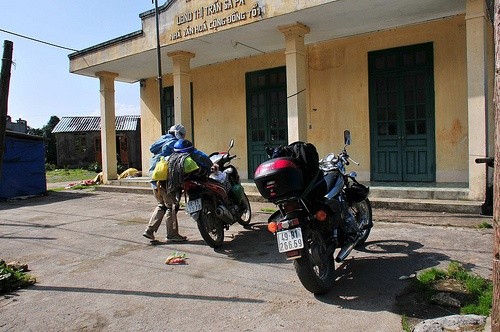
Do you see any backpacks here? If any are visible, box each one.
[265,141,321,182]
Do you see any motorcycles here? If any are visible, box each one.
[253,128,374,296]
[181,138,251,248]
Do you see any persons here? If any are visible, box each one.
[142,124,186,241]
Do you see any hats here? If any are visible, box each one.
[168,124,187,140]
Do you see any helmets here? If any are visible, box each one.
[343,183,371,204]
[173,139,194,154]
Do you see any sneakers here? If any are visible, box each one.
[165,233,187,243]
[143,227,155,241]
